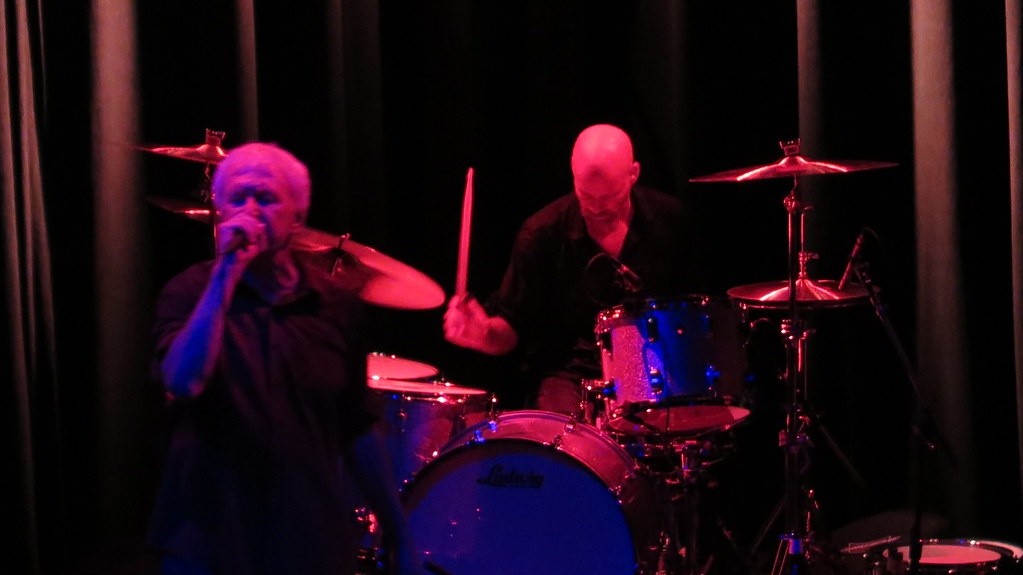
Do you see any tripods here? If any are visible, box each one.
[657,176,849,575]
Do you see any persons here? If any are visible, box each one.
[149,138,358,575]
[443,122,705,409]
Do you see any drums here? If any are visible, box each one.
[594,294,753,437]
[366,350,441,379]
[397,410,663,575]
[367,379,489,517]
[841,537,1023,574]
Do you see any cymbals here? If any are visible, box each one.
[288,224,447,310]
[726,279,881,311]
[686,157,901,182]
[131,142,229,166]
[145,197,214,227]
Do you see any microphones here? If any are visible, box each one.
[838,231,864,291]
[222,232,247,251]
